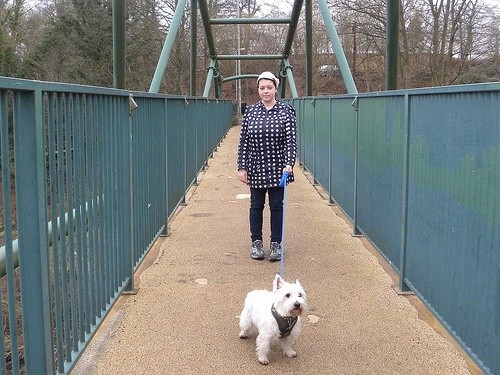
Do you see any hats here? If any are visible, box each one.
[257,71,279,88]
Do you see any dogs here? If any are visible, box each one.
[238,273,307,365]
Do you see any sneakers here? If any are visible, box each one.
[252,239,265,259]
[269,241,282,261]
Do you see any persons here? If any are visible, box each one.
[237,70,296,261]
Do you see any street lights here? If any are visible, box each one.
[235,47,247,116]
